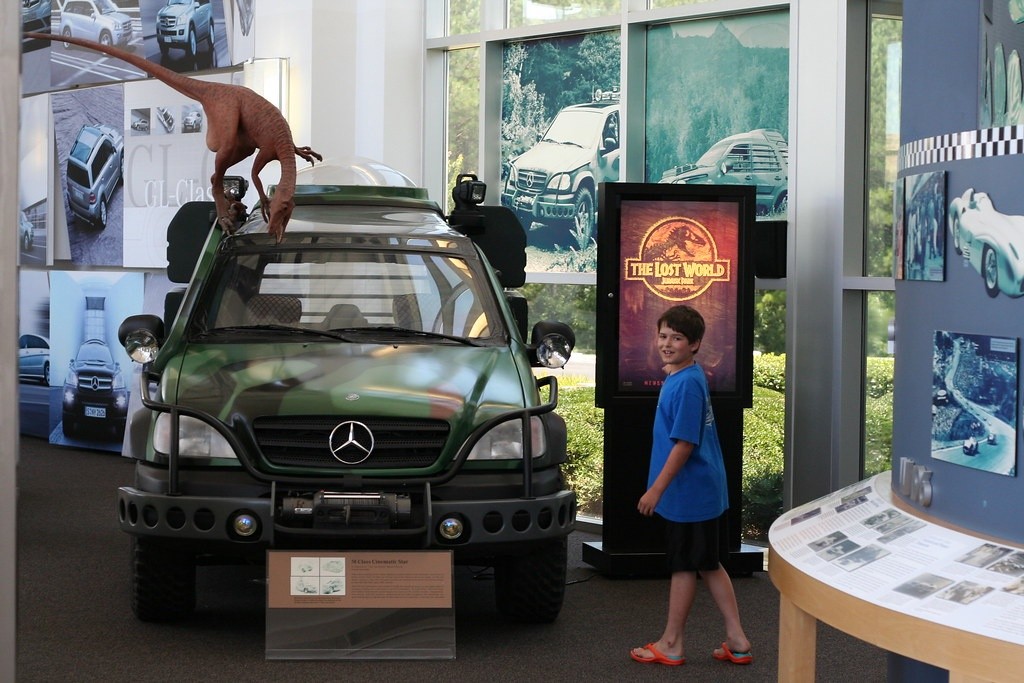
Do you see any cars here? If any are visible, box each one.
[18,332,50,389]
[21,2,52,51]
[986,433,998,445]
[964,436,978,455]
[948,187,1024,299]
[20,210,35,251]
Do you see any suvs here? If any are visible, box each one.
[655,126,789,216]
[60,1,133,58]
[62,339,131,442]
[131,118,150,131]
[502,86,621,251]
[156,1,215,58]
[117,182,576,624]
[65,123,124,230]
[183,110,203,133]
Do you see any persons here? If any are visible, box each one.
[629,304,753,666]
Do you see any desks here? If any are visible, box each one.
[767,468,1024,682]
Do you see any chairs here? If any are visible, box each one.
[243,294,302,327]
[320,304,367,328]
[392,293,453,336]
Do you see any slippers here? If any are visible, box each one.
[630,643,685,666]
[713,642,753,663]
